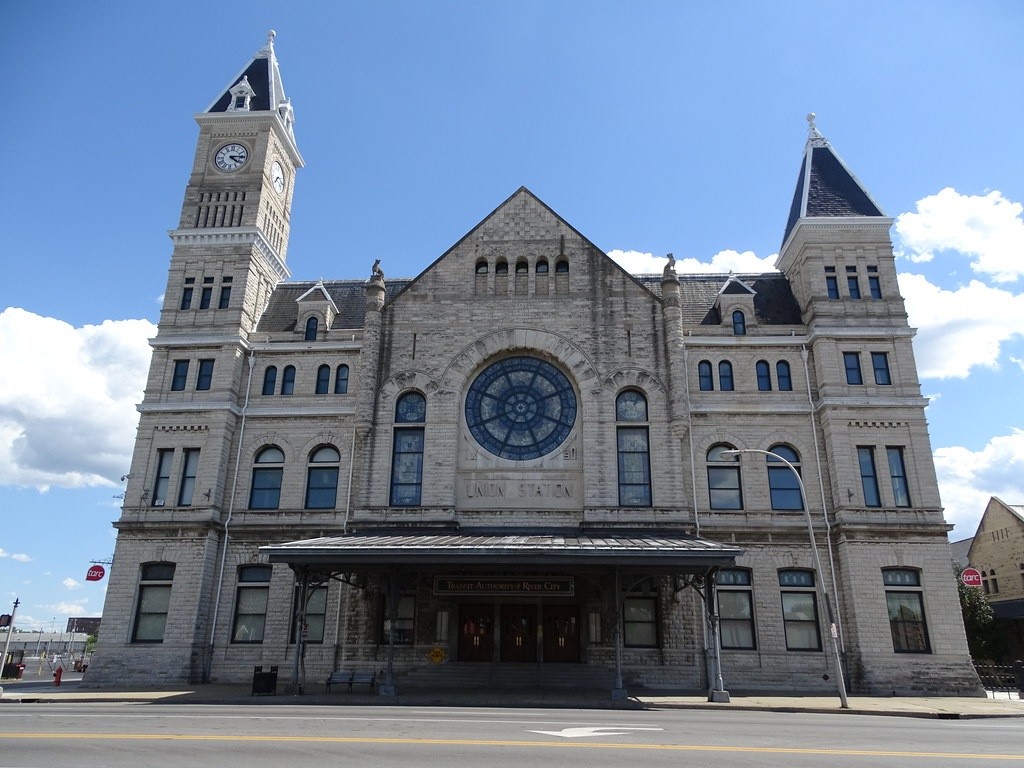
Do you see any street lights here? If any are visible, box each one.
[719,449,850,708]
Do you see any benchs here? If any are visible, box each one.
[324,672,376,696]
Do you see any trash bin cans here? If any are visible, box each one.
[250,665,279,696]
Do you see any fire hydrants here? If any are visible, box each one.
[52,666,66,687]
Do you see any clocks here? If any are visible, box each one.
[266,154,288,201]
[210,140,252,174]
